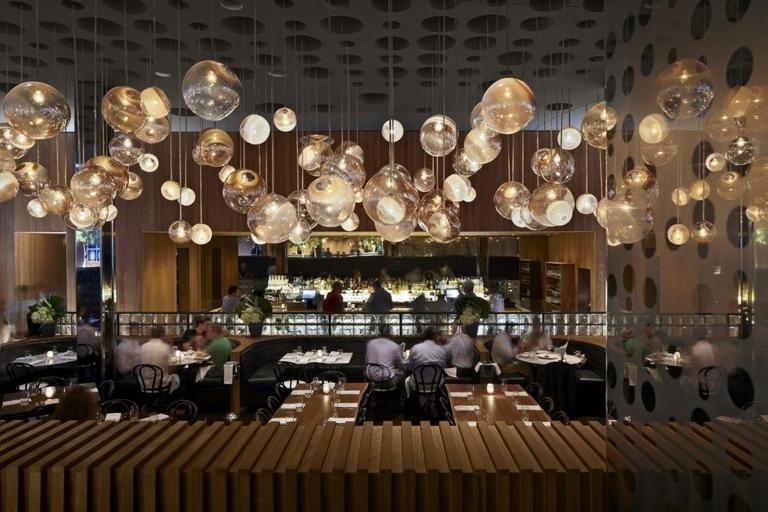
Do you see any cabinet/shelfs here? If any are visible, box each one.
[544,261,576,313]
[519,259,542,311]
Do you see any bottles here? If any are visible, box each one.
[348,237,381,252]
[263,272,486,300]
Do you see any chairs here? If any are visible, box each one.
[5,343,198,426]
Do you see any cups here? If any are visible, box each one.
[172,346,201,364]
[292,346,342,361]
[405,349,410,359]
[285,376,345,424]
[545,344,560,356]
[462,381,527,422]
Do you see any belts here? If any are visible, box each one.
[369,376,393,384]
[417,381,440,385]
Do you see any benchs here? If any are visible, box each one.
[117,334,237,417]
[235,334,484,407]
[482,336,606,415]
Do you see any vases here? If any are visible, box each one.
[40,324,56,338]
[462,323,479,338]
[248,323,262,338]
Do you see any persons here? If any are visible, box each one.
[624,324,742,392]
[323,281,344,312]
[79,315,99,360]
[361,277,393,312]
[223,285,239,312]
[51,384,99,421]
[115,319,139,375]
[365,278,553,400]
[4,284,38,338]
[138,327,180,402]
[177,314,211,354]
[242,289,273,316]
[191,323,232,377]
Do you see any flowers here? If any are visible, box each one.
[456,296,490,326]
[29,296,65,324]
[235,293,273,324]
